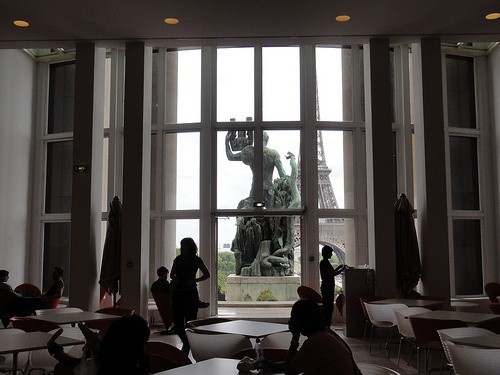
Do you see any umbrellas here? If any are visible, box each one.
[99,195,122,305]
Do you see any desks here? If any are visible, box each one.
[406,310,500,375]
[190,320,289,349]
[0,331,85,375]
[368,298,448,344]
[23,311,124,352]
[150,358,285,375]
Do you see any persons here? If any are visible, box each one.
[48,317,150,375]
[170,238,210,356]
[151,266,210,308]
[0,267,64,317]
[254,299,355,375]
[320,245,347,328]
[225,130,297,197]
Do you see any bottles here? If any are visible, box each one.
[254,338,265,371]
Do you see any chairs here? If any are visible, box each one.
[0,283,500,375]
